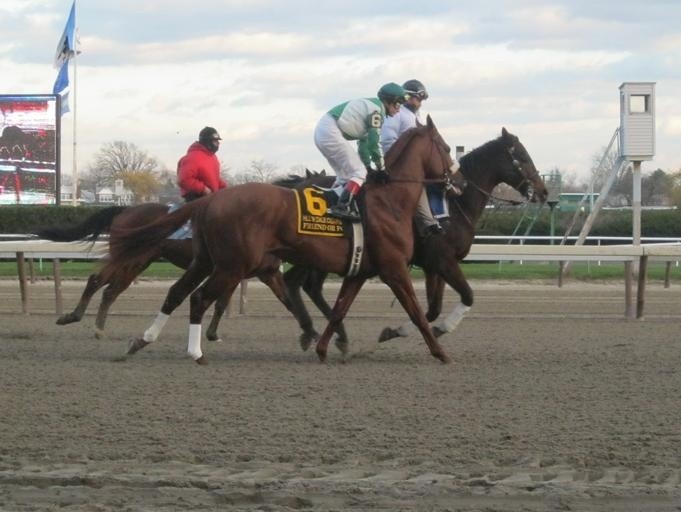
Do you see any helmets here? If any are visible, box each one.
[402,79,427,96]
[199,126,222,142]
[377,83,406,103]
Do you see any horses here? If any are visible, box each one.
[280,129,549,342]
[96,115,464,364]
[28,167,337,333]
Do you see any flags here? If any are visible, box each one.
[50,2,77,119]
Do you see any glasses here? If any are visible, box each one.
[414,91,430,100]
[389,99,401,110]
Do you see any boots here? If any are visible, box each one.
[331,191,362,224]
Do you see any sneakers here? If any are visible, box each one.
[426,220,450,239]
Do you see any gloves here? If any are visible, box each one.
[376,171,391,185]
[366,170,377,178]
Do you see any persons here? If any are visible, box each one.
[175,126,228,204]
[376,80,446,248]
[312,81,406,222]
